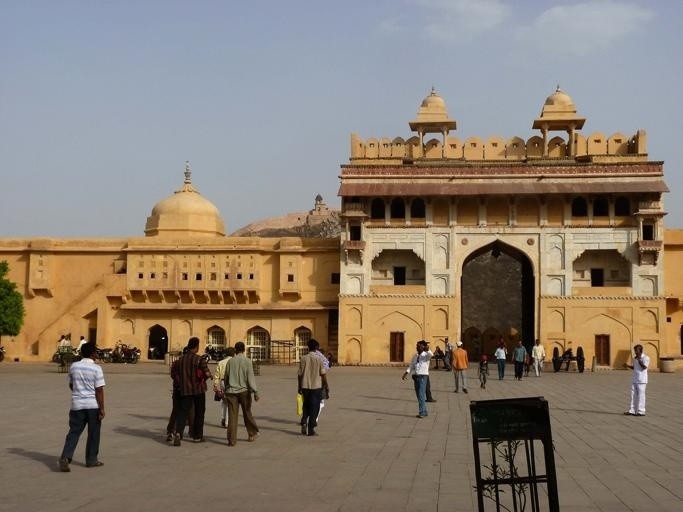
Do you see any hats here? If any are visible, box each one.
[456,341,463,347]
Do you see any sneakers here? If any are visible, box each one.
[416,412,430,419]
[299,420,318,436]
[58,457,71,473]
[249,431,261,441]
[227,439,237,448]
[85,460,105,468]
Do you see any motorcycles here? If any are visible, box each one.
[201,344,235,362]
[0,346,6,364]
[48,347,143,365]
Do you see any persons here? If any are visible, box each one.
[402,336,546,418]
[57,334,141,366]
[166,337,262,447]
[622,345,650,416]
[306,341,329,436]
[59,342,106,471]
[297,341,330,436]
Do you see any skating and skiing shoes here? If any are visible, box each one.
[165,426,208,447]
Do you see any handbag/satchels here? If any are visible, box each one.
[296,394,304,418]
